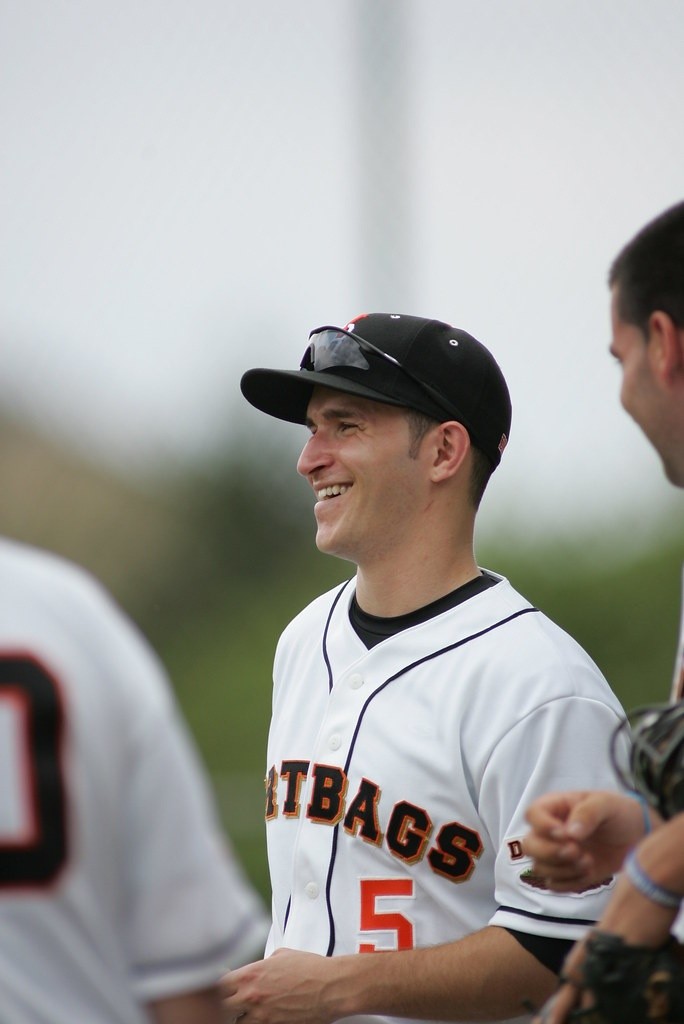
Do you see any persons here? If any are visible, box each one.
[0,536,272,1023]
[220,311,644,1024]
[522,198,684,1024]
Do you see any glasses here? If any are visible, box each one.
[301,327,467,429]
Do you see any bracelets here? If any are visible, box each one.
[624,853,681,910]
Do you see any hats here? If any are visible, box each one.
[241,313,512,466]
